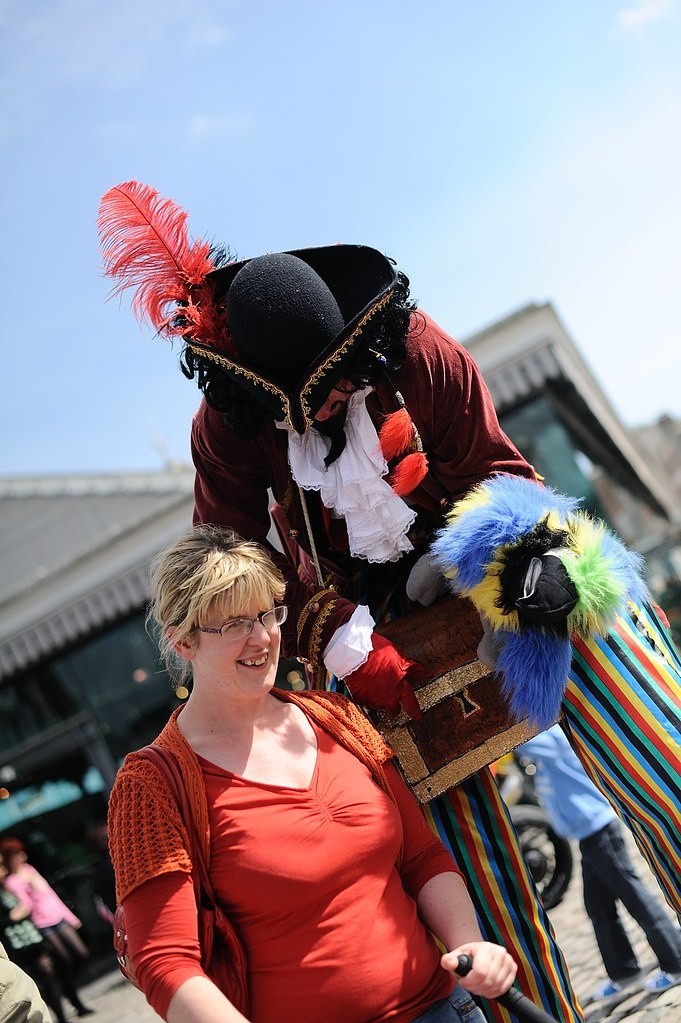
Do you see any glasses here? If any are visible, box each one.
[196,606,288,643]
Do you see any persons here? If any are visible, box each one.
[175,244,680,1023]
[512,717,680,998]
[0,838,93,1023]
[108,526,518,1023]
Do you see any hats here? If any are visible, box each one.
[95,179,399,435]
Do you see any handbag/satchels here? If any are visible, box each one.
[113,744,247,1023]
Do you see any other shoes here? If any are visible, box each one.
[644,971,681,992]
[592,972,642,1000]
[77,1006,93,1017]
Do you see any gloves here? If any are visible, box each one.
[515,546,581,623]
[322,604,426,722]
